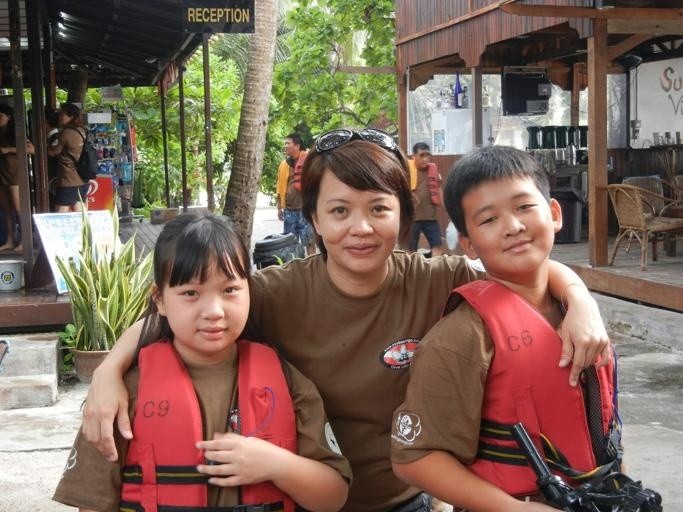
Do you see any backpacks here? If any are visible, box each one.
[61,126,97,182]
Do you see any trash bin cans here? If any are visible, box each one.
[255,232,299,270]
[551,189,582,243]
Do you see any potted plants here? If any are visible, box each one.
[54,187,156,383]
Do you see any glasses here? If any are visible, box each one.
[315,128,397,156]
[419,152,431,158]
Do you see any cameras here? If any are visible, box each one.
[510,421,663,512]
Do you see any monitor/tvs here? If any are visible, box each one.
[503,65,552,115]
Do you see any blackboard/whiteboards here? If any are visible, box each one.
[33,210,121,295]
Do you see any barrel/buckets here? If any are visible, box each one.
[0,247,27,293]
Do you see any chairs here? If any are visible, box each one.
[607,175,683,269]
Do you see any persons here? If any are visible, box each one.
[47,104,88,213]
[0,104,35,251]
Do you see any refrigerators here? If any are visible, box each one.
[53,174,114,218]
